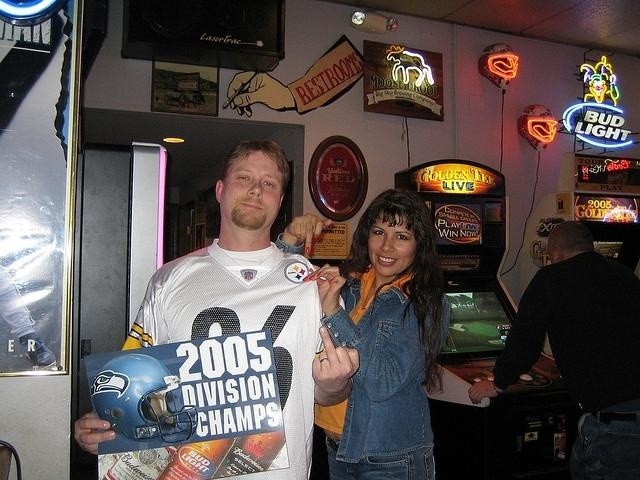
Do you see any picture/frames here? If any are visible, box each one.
[0,0,85,377]
[151,60,220,117]
[308,135,368,222]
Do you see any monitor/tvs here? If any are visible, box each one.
[433,277,524,365]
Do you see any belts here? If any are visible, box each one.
[592,411,637,424]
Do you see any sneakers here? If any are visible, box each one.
[20,333,56,366]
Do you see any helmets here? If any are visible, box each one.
[90,353,198,443]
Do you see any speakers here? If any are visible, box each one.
[119,0,288,74]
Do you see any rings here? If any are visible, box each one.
[319,355,328,362]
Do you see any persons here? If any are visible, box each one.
[227,33,367,117]
[75,137,359,480]
[276,187,450,480]
[0,265,59,371]
[466,221,640,479]
